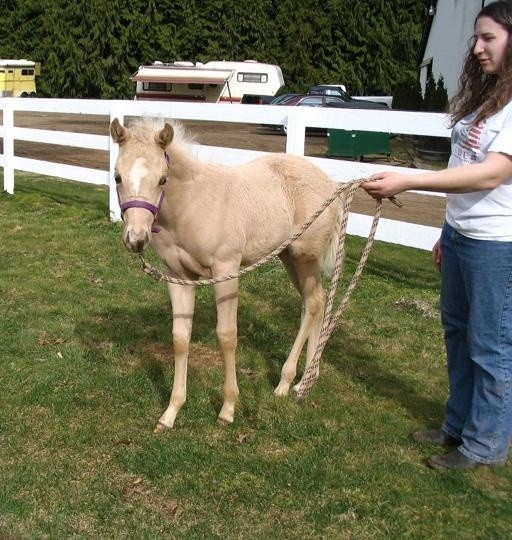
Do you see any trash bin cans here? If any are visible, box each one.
[327,101,391,162]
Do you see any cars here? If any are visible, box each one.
[261,84,393,137]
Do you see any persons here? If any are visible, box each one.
[359,0,512,472]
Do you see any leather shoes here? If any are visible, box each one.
[413,430,506,471]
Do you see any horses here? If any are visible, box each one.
[106,113,342,437]
